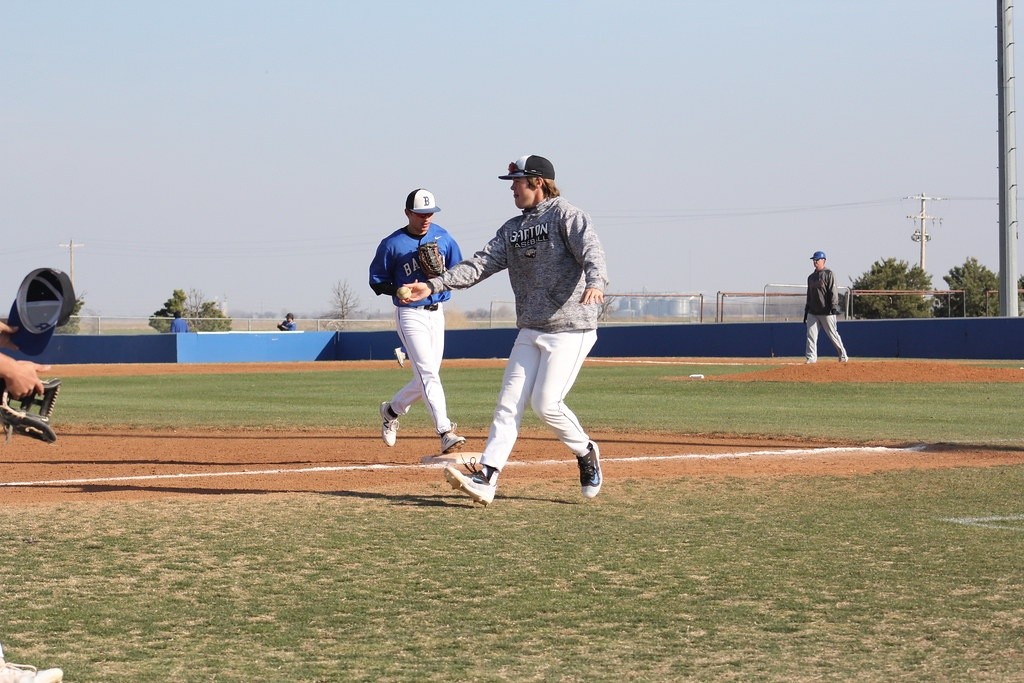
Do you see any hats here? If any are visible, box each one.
[498,155,555,180]
[285,313,293,318]
[406,189,441,214]
[6,268,75,355]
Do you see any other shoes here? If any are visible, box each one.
[839,357,849,363]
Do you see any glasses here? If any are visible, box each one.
[508,164,544,177]
[409,210,434,219]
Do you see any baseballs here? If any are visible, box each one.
[397,286,412,300]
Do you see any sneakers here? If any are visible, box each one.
[441,423,466,454]
[443,458,498,508]
[380,402,399,447]
[579,439,602,499]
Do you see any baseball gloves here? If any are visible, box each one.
[0,375,62,440]
[417,241,445,278]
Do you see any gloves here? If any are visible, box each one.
[803,312,809,324]
[832,309,836,314]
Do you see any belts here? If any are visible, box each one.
[424,304,439,311]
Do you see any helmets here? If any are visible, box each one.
[810,252,828,261]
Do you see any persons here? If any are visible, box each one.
[277,313,296,332]
[170,311,189,333]
[0,321,54,403]
[397,155,609,506]
[368,188,467,454]
[804,251,848,364]
[395,343,406,368]
